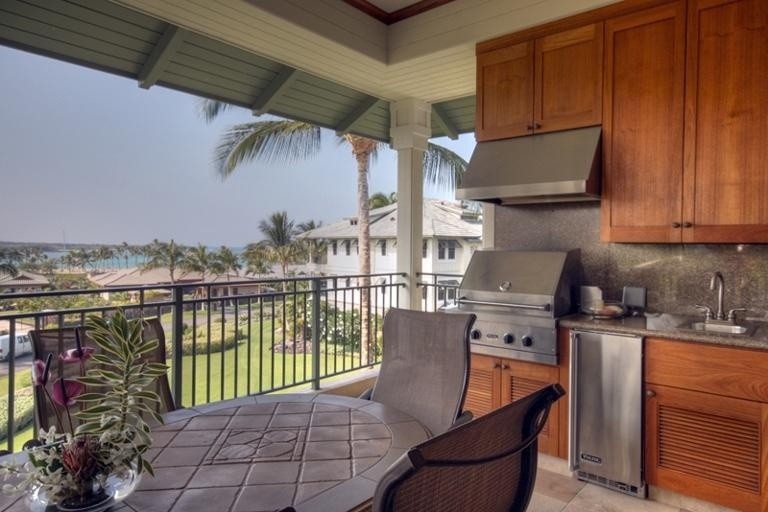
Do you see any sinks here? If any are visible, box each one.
[675,319,756,339]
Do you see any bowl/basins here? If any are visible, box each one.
[581,298,630,320]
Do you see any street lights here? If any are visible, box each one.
[297,270,314,349]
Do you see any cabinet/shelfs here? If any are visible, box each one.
[461,329,570,460]
[471,21,603,143]
[601,1,768,244]
[642,336,768,512]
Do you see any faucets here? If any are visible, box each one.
[709,271,726,320]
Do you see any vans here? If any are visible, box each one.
[0,331,33,360]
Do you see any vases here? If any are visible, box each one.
[23,457,144,512]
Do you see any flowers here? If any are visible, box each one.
[0,304,169,497]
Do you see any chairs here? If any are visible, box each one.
[23,317,177,452]
[355,306,477,431]
[371,383,566,512]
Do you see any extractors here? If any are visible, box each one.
[453,123,603,207]
